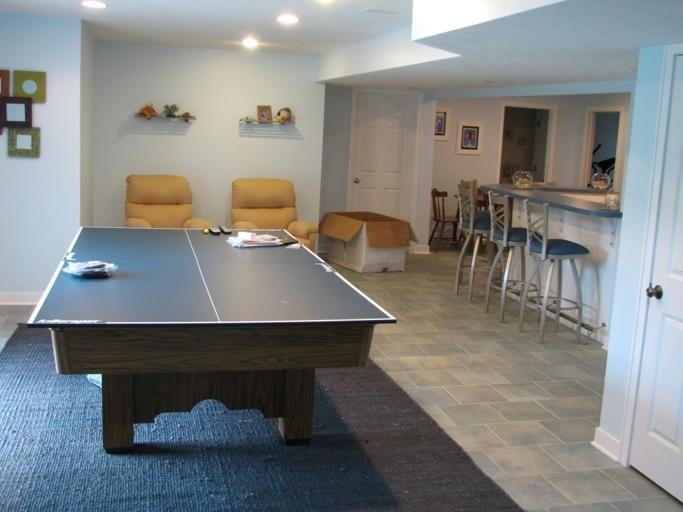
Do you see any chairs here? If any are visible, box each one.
[485,190,544,324]
[122,172,214,231]
[428,187,458,251]
[517,199,593,345]
[228,176,319,252]
[453,178,495,304]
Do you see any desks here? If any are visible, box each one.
[454,188,508,215]
[26,223,402,455]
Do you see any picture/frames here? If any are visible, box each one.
[0,64,49,159]
[456,120,484,155]
[435,107,451,142]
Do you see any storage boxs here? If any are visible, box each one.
[316,210,420,276]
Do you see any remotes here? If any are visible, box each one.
[219,225,231,234]
[208,226,221,234]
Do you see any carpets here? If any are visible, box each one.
[0,321,533,511]
